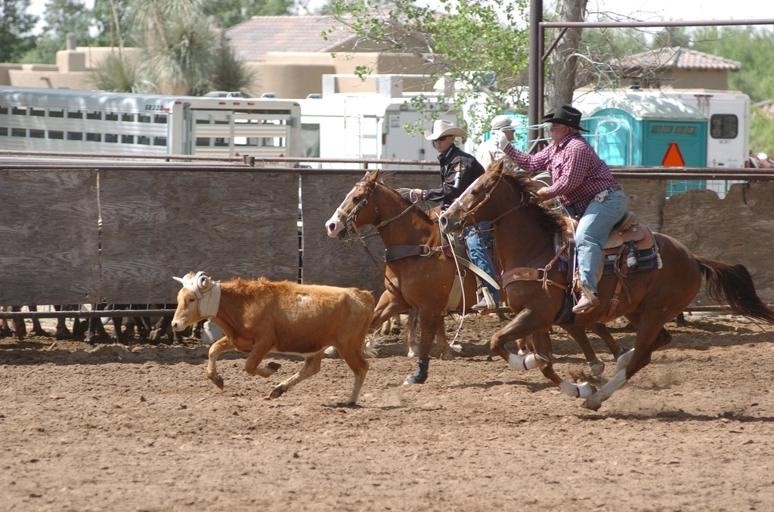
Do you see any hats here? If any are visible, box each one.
[491,115,520,134]
[423,120,467,141]
[540,105,591,133]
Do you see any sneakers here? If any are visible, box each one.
[571,291,598,314]
[471,293,500,311]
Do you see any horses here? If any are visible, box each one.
[436,157,774,413]
[320,166,626,387]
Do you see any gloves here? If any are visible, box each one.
[492,129,509,151]
[409,188,423,201]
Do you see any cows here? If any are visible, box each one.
[171,270,375,406]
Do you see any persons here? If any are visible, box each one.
[407,119,503,312]
[490,103,629,316]
[474,114,517,176]
